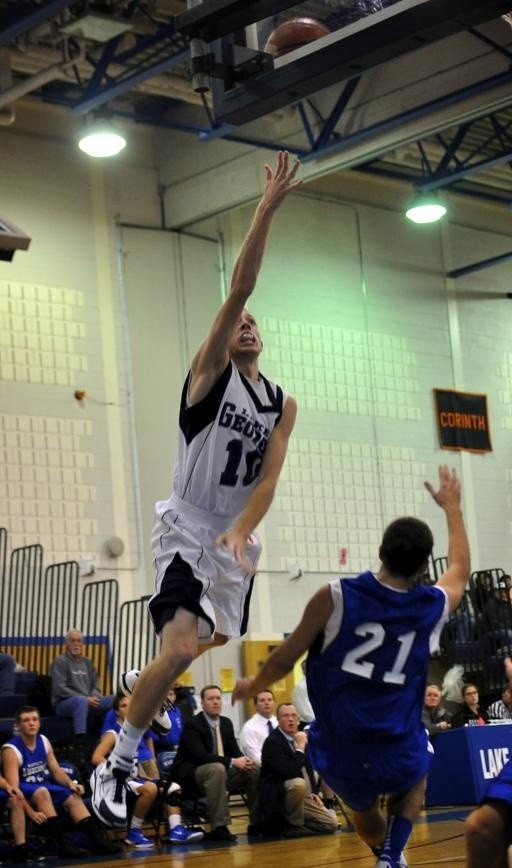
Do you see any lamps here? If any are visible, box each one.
[403,177,449,227]
[77,107,127,159]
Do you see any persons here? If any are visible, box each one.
[421,681,511,736]
[232,466,471,868]
[90,151,303,828]
[0,630,339,868]
[463,759,511,868]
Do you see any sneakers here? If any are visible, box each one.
[90,754,130,829]
[0,842,46,866]
[119,667,174,737]
[373,854,402,868]
[85,836,124,856]
[168,825,205,843]
[55,841,93,859]
[124,829,157,849]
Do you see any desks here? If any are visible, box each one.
[424,723,512,807]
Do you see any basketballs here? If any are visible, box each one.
[264,17,330,58]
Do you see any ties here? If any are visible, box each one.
[212,724,227,758]
[266,720,275,736]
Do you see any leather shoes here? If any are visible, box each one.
[212,826,238,841]
